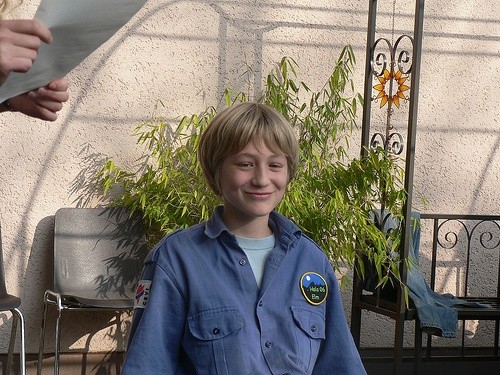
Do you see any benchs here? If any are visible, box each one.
[350,212,500,374]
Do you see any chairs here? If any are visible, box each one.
[37,207,149,375]
[0,221,26,375]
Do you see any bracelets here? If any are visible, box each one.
[2,100,15,112]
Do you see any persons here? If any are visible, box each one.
[121,103,367,375]
[0,19,70,122]
